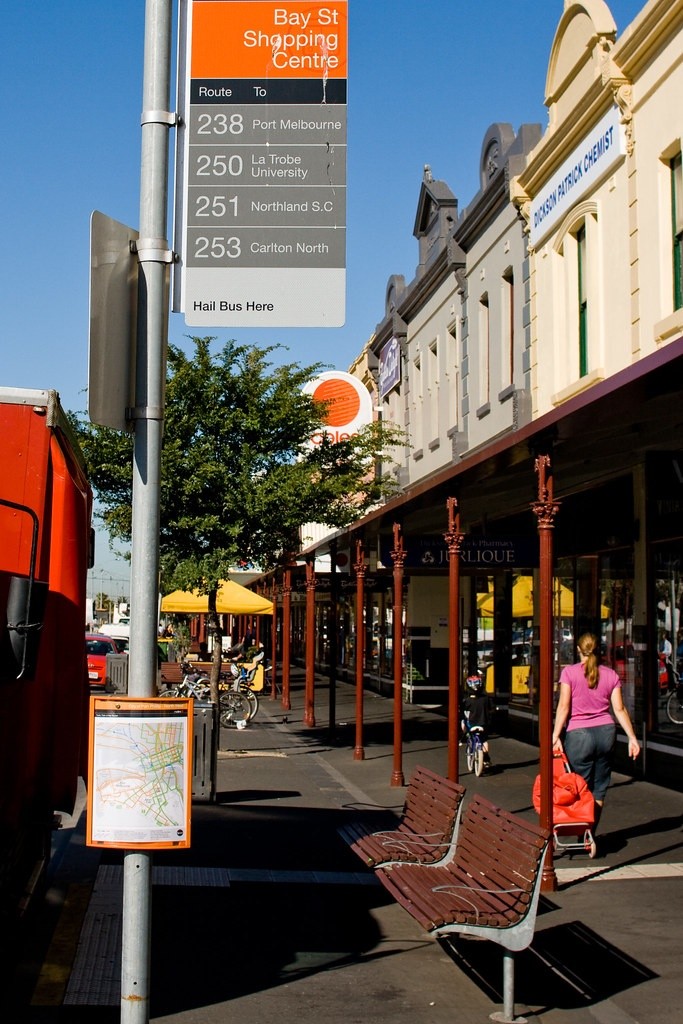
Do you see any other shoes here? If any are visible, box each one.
[484,760,492,768]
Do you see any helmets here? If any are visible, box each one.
[464,676,483,695]
[231,663,240,679]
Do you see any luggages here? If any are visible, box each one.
[532,750,596,860]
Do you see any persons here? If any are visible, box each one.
[196,635,251,661]
[657,633,676,691]
[551,632,640,844]
[161,622,177,637]
[621,634,634,657]
[460,675,493,767]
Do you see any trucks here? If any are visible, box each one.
[1,381,96,931]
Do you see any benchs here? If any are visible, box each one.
[335,763,468,868]
[375,794,552,1024]
[161,662,236,697]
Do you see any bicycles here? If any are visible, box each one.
[666,667,683,726]
[458,712,489,777]
[158,651,265,729]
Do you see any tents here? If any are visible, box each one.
[160,577,273,654]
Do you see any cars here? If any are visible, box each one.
[603,641,670,696]
[85,624,131,691]
[463,625,576,675]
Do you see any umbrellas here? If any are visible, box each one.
[476,576,609,643]
